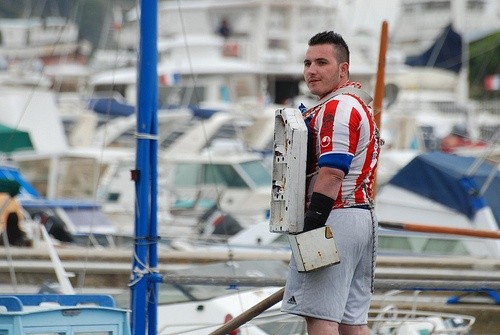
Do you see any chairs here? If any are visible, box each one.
[0,293,133,335]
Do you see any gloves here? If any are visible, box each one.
[302,192,336,233]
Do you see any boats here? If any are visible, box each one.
[0,0,500,335]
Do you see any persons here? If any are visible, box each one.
[279,30,383,335]
[1,178,75,246]
